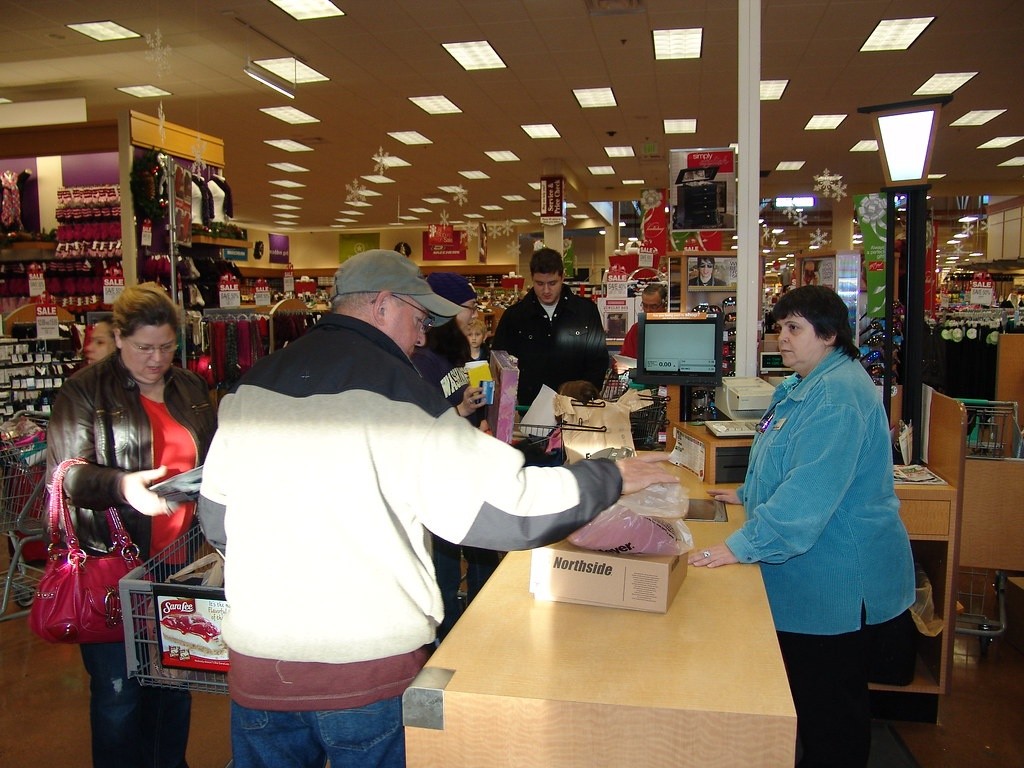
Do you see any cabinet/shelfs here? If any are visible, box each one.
[665,251,738,422]
[760,351,795,371]
[866,462,956,696]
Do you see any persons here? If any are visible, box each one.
[197,251,680,768]
[47,284,216,768]
[689,256,726,286]
[620,282,668,360]
[408,272,499,642]
[687,284,916,768]
[192,173,214,226]
[206,174,233,223]
[466,319,488,366]
[525,380,601,467]
[83,314,120,367]
[491,247,610,422]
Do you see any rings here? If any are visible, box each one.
[704,550,710,557]
[469,400,472,403]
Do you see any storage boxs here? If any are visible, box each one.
[764,334,780,352]
[486,350,520,445]
[528,539,689,615]
[597,297,634,339]
[318,276,335,287]
[607,281,628,298]
[768,377,785,387]
[501,278,524,290]
[634,296,645,324]
[295,281,317,299]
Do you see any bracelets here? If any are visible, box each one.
[484,429,494,436]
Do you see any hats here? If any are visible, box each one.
[331,249,463,328]
[426,272,478,304]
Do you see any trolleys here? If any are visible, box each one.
[952,397,1024,660]
[0,408,54,621]
[512,365,674,457]
[117,520,237,768]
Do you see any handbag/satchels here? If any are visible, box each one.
[27,457,156,644]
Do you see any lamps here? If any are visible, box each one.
[958,213,983,223]
[230,15,307,100]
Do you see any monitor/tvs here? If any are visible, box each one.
[636,313,724,422]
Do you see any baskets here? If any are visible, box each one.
[118,522,230,695]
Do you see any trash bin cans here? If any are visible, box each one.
[868,569,931,686]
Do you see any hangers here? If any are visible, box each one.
[205,312,269,322]
[277,308,326,315]
[941,311,1001,330]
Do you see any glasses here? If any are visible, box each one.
[460,305,479,315]
[370,292,434,327]
[124,336,180,356]
[859,301,904,385]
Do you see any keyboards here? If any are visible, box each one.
[705,420,762,439]
